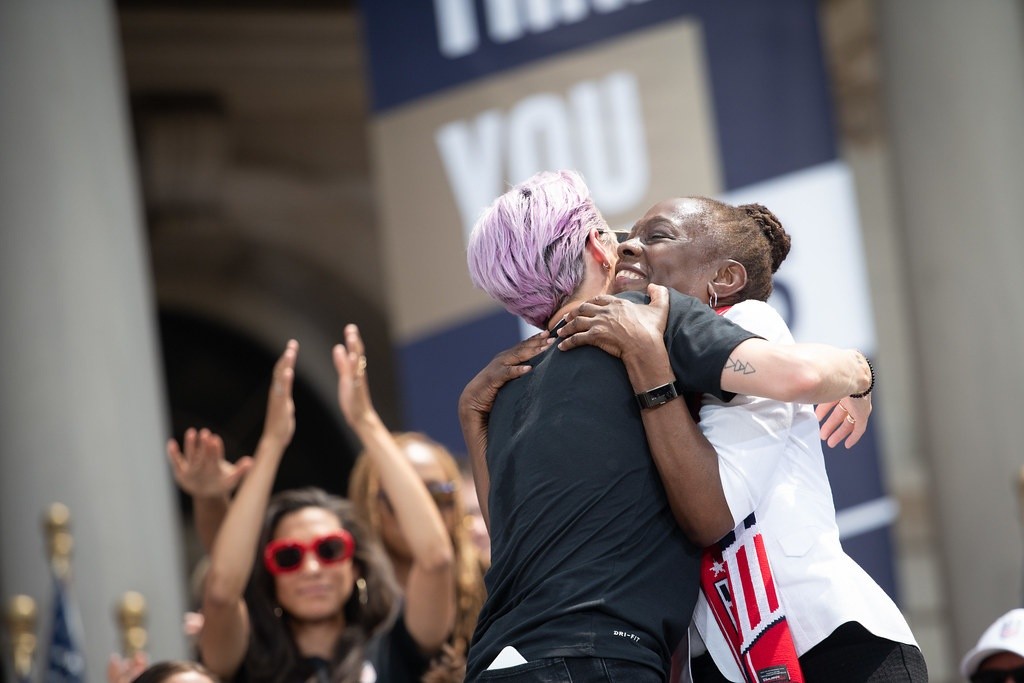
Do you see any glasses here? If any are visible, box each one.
[975,666,1024,683]
[263,528,356,576]
[584,228,632,247]
[375,479,456,515]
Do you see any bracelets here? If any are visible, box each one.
[849,355,875,398]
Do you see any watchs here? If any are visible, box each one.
[634,380,684,411]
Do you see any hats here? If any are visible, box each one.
[960,608,1024,680]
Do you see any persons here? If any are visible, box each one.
[456,168,929,683]
[0,323,491,683]
[959,608,1024,683]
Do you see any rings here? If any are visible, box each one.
[839,401,847,411]
[846,413,855,424]
